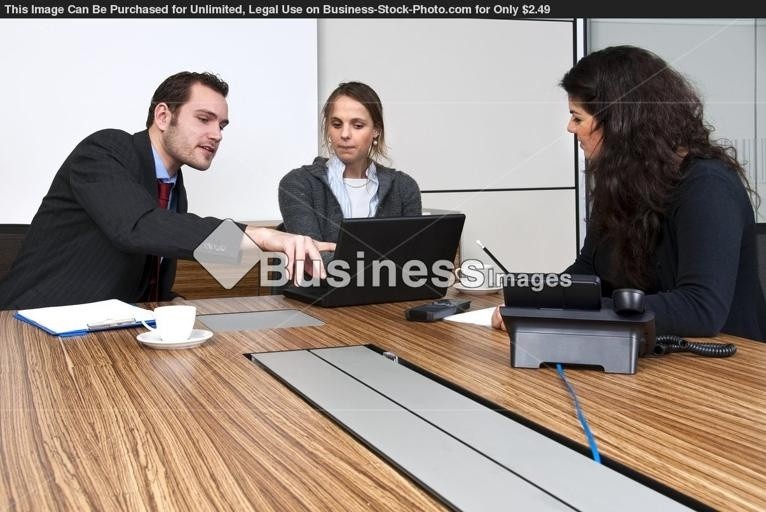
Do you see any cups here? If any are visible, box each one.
[140,306,197,343]
[456,264,494,289]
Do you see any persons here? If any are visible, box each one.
[278,81,422,290]
[0,71,338,311]
[492,45,766,343]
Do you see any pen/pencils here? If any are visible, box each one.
[476,239,509,274]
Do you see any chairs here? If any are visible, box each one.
[271,220,294,296]
[1,223,33,309]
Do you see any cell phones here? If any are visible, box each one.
[404,298,472,322]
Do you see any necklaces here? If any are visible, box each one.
[344,181,371,188]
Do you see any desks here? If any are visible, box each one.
[1,287,765,511]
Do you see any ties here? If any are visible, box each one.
[158,181,174,210]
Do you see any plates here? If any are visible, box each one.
[454,283,503,296]
[136,330,213,350]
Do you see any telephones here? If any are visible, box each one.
[499,272,658,374]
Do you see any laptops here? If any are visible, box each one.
[281,214,466,307]
